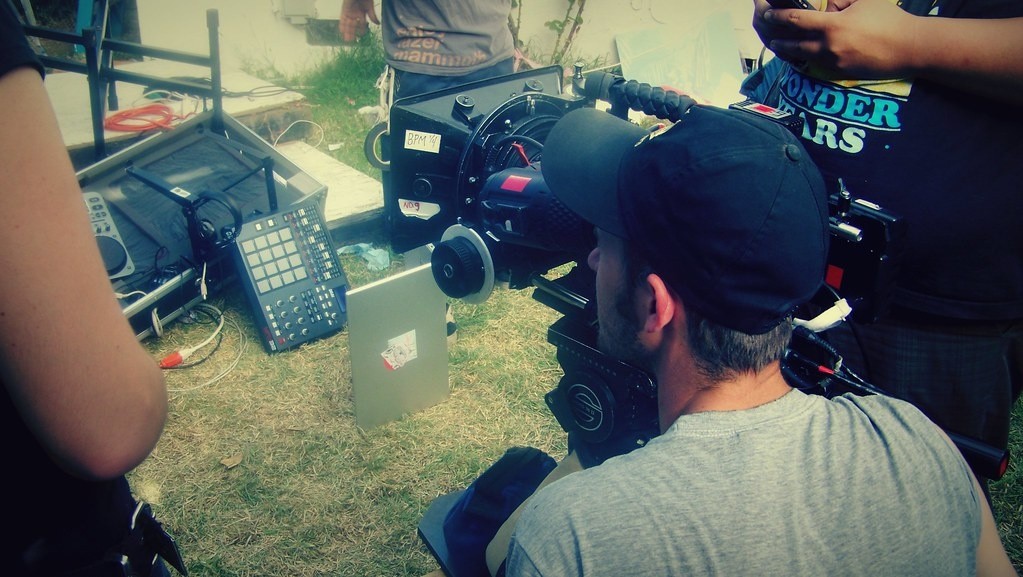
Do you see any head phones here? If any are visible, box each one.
[185,190,243,253]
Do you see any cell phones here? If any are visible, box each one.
[765,0,816,10]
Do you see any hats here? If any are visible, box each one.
[541,103,830,335]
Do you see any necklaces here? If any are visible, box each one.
[484,104,1014,576]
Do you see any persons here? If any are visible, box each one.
[338,0,515,206]
[0,0,173,577]
[743,1,1022,449]
